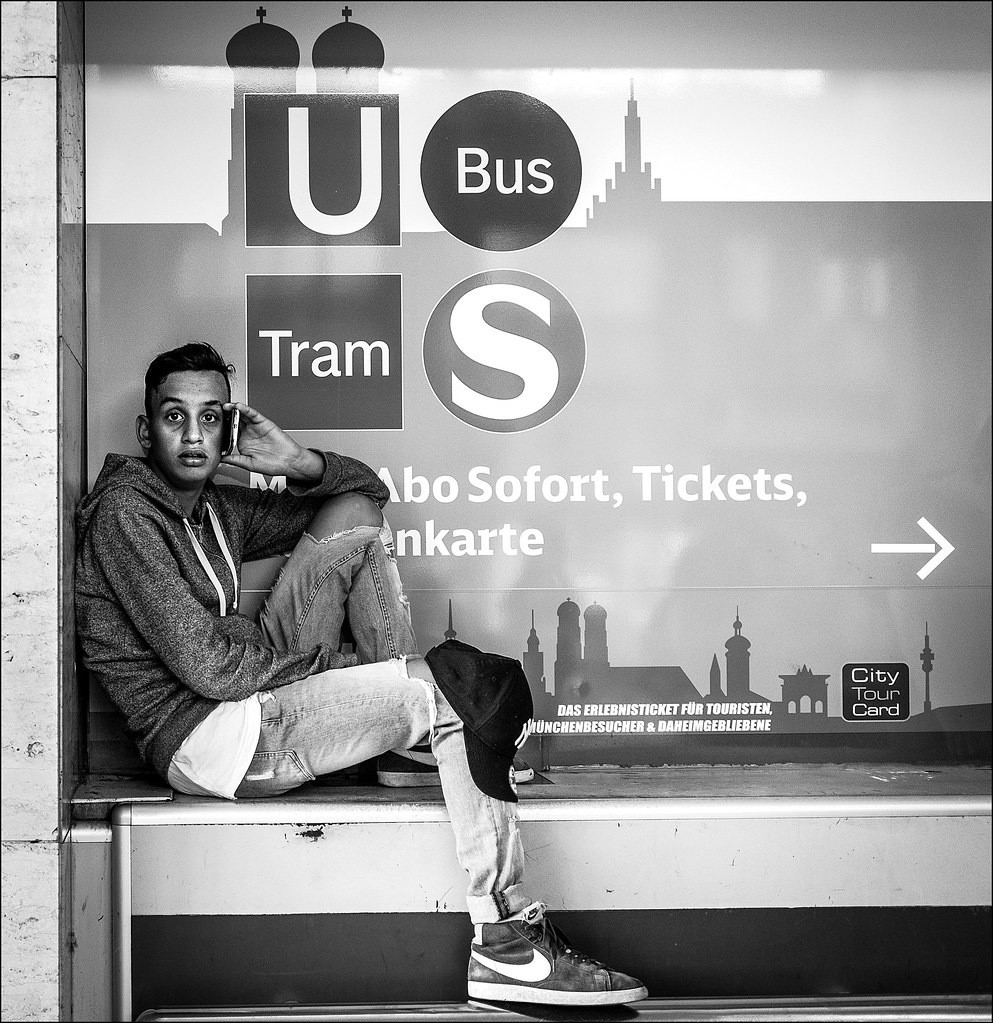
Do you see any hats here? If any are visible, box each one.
[424,639,534,803]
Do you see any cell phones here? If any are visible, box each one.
[221,408,240,456]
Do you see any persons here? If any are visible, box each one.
[75,344,647,1004]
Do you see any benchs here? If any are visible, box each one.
[111,768,993,1023]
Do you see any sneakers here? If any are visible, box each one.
[376,744,536,787]
[468,917,649,1005]
